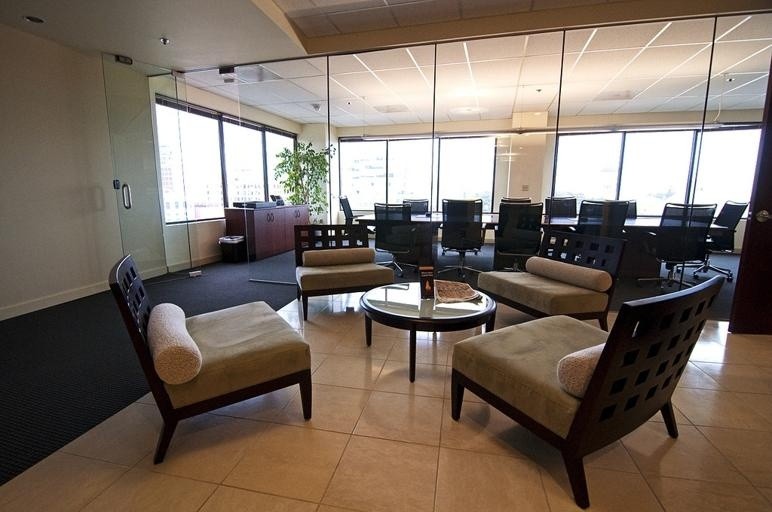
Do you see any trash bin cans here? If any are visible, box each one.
[219,236,245,263]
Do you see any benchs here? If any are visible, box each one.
[477,229,627,333]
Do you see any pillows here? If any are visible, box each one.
[148,301,202,387]
[302,247,375,266]
[526,256,613,292]
[555,340,606,397]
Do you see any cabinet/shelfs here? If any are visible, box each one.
[225,203,310,262]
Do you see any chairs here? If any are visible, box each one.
[109,253,312,463]
[294,223,395,320]
[451,273,726,510]
